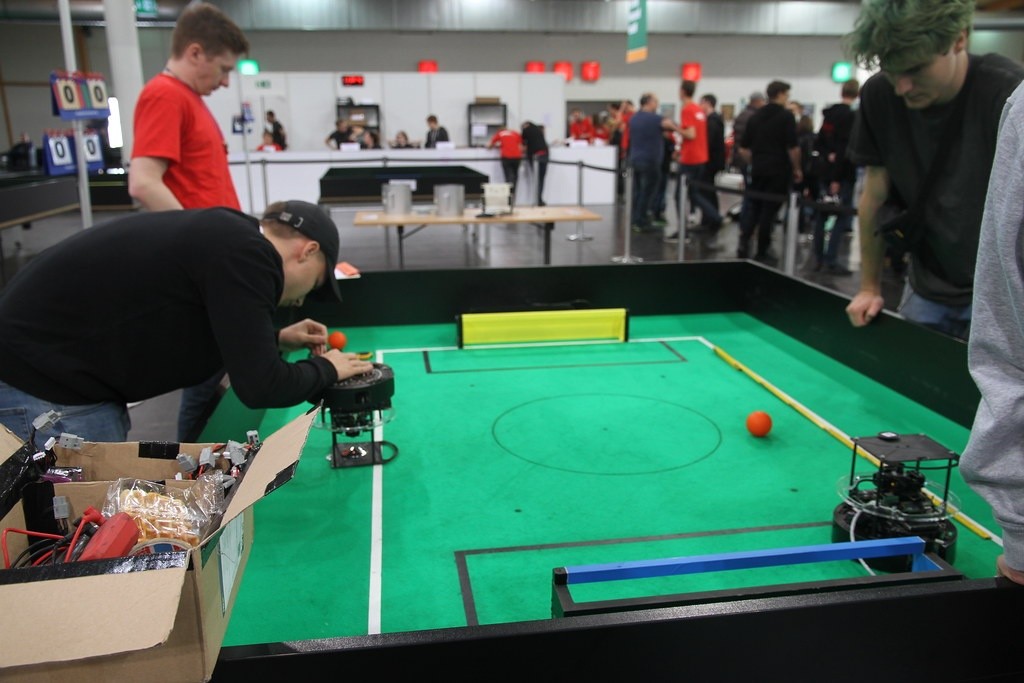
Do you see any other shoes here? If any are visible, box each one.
[662,232,692,244]
[828,227,853,236]
[631,220,643,234]
[827,263,853,276]
[699,222,724,240]
[690,224,710,233]
[652,218,668,226]
[810,260,824,273]
[538,202,546,206]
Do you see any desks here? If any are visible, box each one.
[318,164,488,267]
[354,203,604,269]
[197,259,1023,683]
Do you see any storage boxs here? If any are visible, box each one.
[0,399,324,683]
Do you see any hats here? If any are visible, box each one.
[262,201,343,303]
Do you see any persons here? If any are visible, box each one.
[424,115,448,148]
[521,122,549,206]
[732,0,1024,337]
[628,79,726,243]
[958,80,1024,583]
[0,201,375,451]
[128,3,247,445]
[324,120,381,149]
[264,112,287,150]
[487,128,524,205]
[258,132,281,152]
[389,131,421,148]
[569,101,635,204]
